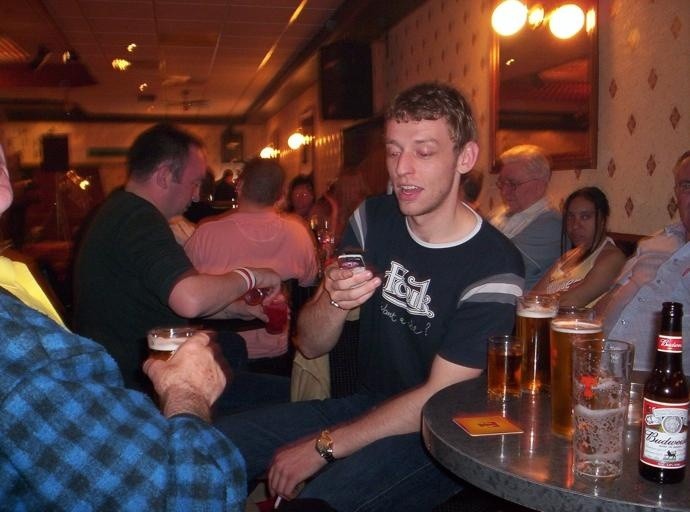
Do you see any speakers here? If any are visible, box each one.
[320,39,373,121]
[43,134,70,174]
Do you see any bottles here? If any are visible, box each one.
[307,212,336,252]
[633,300,689,485]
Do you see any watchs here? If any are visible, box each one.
[314,429,336,464]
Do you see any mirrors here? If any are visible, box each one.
[487,0,599,175]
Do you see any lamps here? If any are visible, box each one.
[492,0,545,35]
[288,134,310,150]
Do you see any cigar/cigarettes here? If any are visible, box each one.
[274,497,281,509]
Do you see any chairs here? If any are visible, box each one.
[606,231,652,257]
[200,280,299,375]
[39,257,71,311]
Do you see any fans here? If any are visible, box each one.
[162,90,212,110]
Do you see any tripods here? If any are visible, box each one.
[31,176,74,247]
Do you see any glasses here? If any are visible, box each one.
[495,179,537,190]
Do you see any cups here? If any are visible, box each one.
[244,286,271,307]
[548,301,605,442]
[486,333,525,401]
[144,324,198,361]
[513,293,560,396]
[260,284,291,336]
[569,334,637,479]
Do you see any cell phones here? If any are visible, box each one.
[337,249,367,276]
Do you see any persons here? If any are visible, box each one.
[284,175,317,217]
[527,186,627,309]
[169,168,238,248]
[486,144,572,273]
[67,119,291,406]
[185,157,318,359]
[594,149,690,374]
[213,82,524,512]
[457,171,487,222]
[0,146,248,512]
